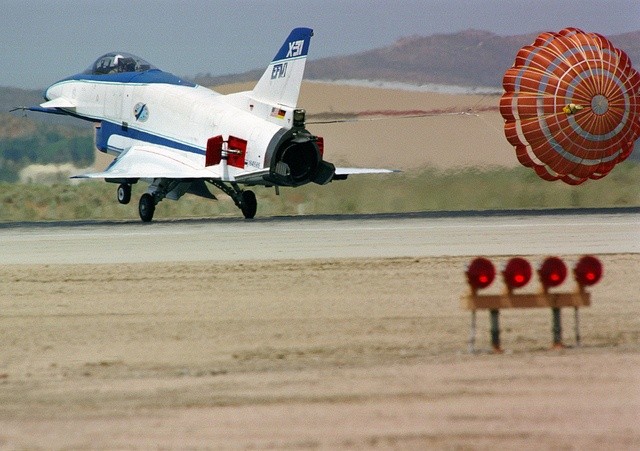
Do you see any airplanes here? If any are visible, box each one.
[9,26,404,225]
